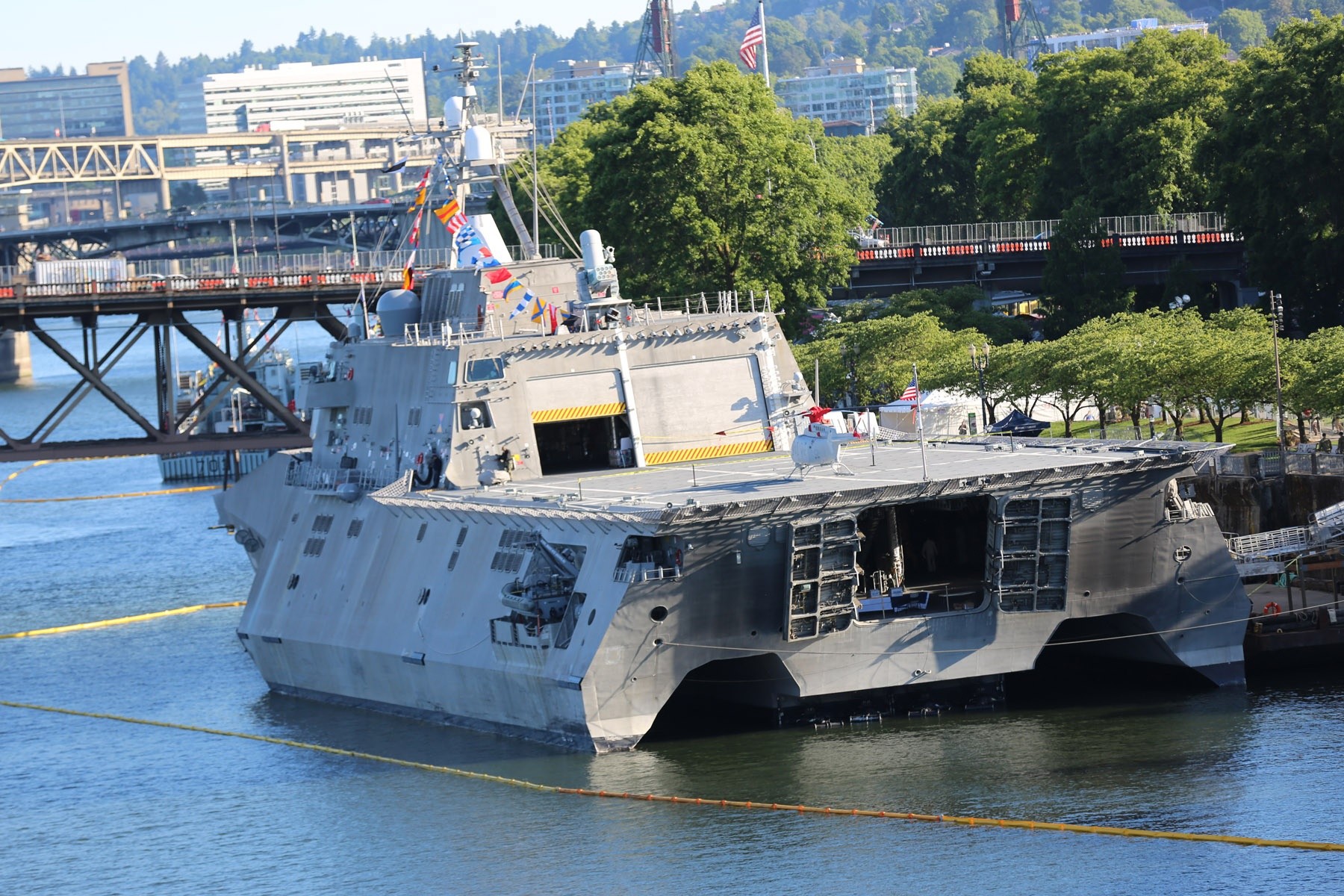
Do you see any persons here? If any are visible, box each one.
[1337,432,1344,454]
[1319,433,1332,454]
[1311,414,1318,435]
[958,421,968,435]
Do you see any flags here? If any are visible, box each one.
[485,267,512,284]
[470,246,492,264]
[446,212,467,234]
[474,259,501,276]
[413,166,429,193]
[548,304,558,335]
[409,208,422,243]
[402,251,415,290]
[406,187,426,212]
[531,297,548,328]
[502,277,523,302]
[451,226,482,263]
[508,289,535,320]
[559,309,580,333]
[900,376,921,401]
[434,199,460,225]
[739,6,764,71]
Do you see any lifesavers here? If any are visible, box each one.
[347,368,354,380]
[417,452,423,464]
[1264,602,1281,618]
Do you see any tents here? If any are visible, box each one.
[987,408,1054,450]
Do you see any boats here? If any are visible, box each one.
[214,42,1344,757]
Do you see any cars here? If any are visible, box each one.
[133,272,189,281]
[154,305,325,481]
[358,196,390,204]
[846,232,889,248]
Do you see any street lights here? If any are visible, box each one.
[1268,290,1287,478]
